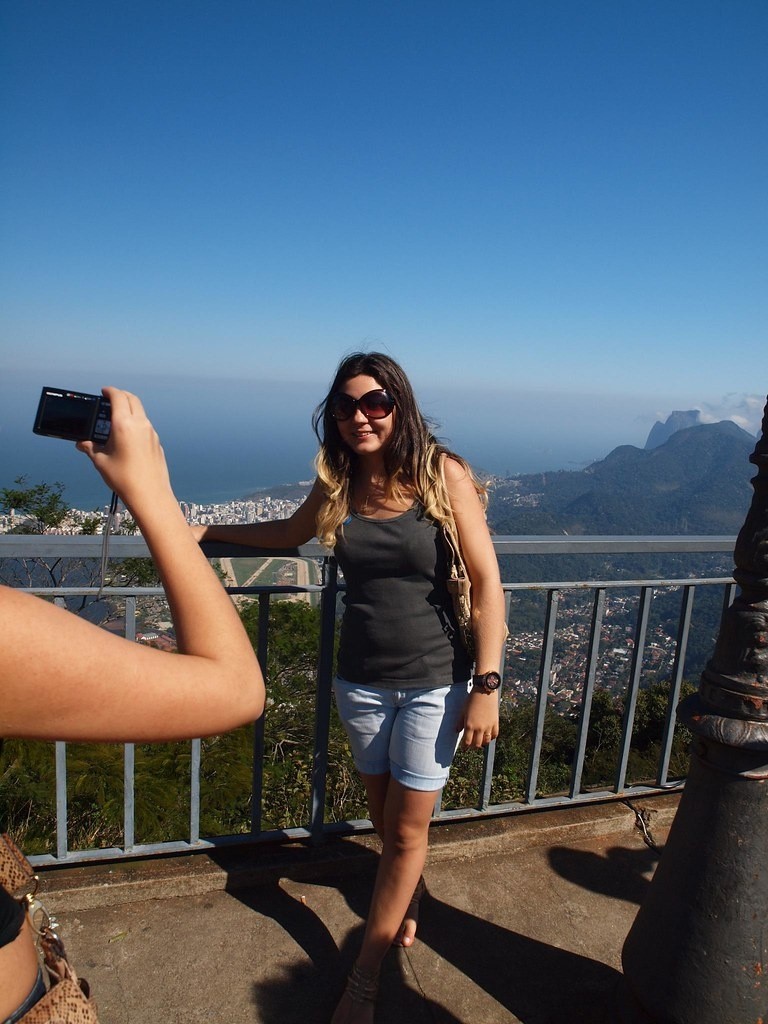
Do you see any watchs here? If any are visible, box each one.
[473,670,501,695]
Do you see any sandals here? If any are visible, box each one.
[393,876,426,947]
[344,965,380,1024]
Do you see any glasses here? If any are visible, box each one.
[328,389,396,422]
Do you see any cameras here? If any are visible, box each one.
[32,385,112,446]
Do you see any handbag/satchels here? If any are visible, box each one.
[14,978,101,1024]
[440,453,509,662]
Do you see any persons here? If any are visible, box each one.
[101,421,107,434]
[190,350,505,1024]
[0,386,266,1024]
